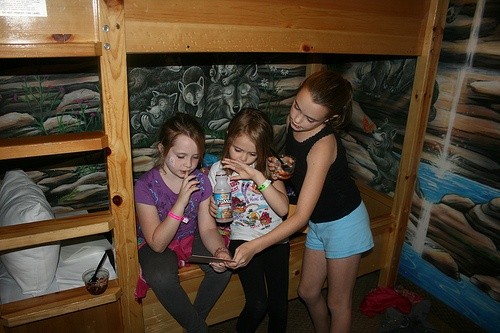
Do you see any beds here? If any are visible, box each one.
[0,0,450,333]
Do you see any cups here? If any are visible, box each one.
[274,155,295,180]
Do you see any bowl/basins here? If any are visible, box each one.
[82,267,109,296]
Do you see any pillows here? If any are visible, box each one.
[0,168,60,295]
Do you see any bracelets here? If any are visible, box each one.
[214,247,232,260]
[167,211,184,221]
[257,179,272,193]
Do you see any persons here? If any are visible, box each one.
[134,112,233,333]
[207,108,291,333]
[225,70,375,333]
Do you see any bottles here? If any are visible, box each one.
[213,169,233,223]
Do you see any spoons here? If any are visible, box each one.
[88,250,107,285]
